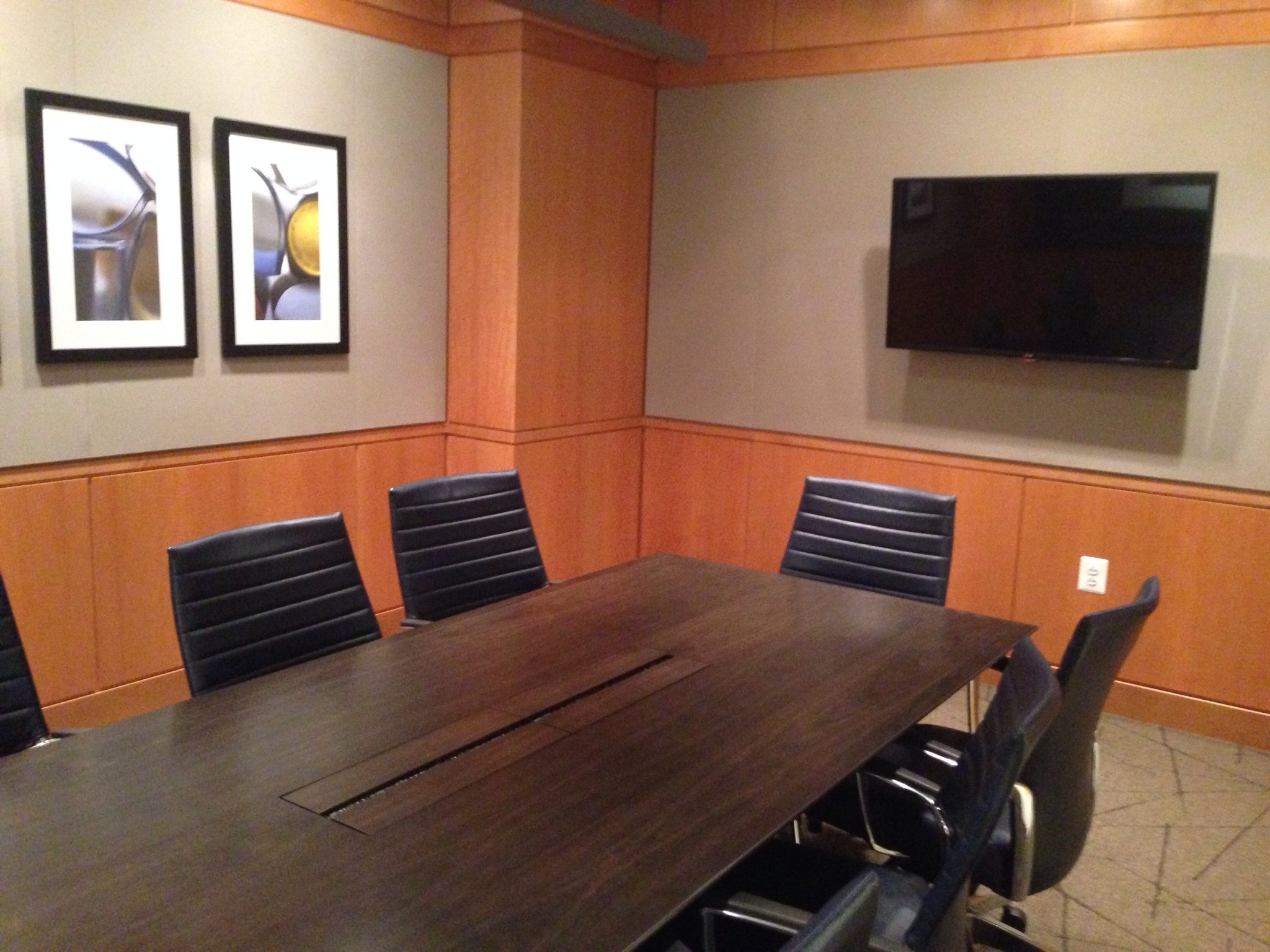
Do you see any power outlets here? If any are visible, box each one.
[1074,555,1109,595]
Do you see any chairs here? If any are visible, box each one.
[168,511,382,699]
[699,636,1060,952]
[387,471,567,633]
[882,575,1162,952]
[782,475,956,603]
[1,570,92,765]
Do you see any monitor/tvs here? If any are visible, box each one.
[885,173,1219,373]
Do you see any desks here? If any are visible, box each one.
[2,545,1043,950]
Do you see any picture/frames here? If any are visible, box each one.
[22,83,201,364]
[210,115,349,359]
